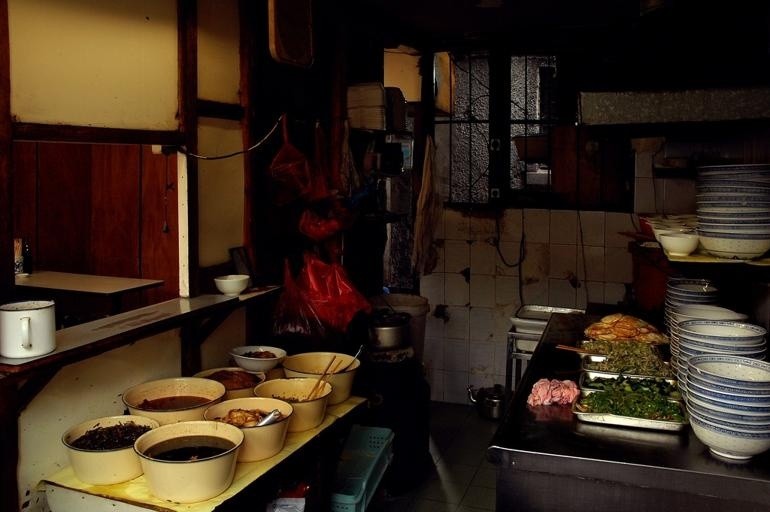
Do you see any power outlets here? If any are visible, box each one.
[491,189,499,197]
[490,138,500,151]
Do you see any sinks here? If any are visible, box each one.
[509,304,586,352]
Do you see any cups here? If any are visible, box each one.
[1,299,58,360]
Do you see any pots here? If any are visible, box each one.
[362,305,414,353]
[512,131,547,161]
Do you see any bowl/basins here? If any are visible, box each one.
[203,394,295,464]
[638,161,768,262]
[252,377,333,433]
[662,276,769,467]
[194,365,267,399]
[59,414,161,487]
[225,343,288,375]
[133,418,245,504]
[282,352,361,406]
[120,377,227,426]
[212,273,252,299]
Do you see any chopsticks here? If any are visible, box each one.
[302,355,344,401]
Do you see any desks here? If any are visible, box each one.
[43,388,372,512]
[486,308,769,509]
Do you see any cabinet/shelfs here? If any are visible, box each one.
[11,137,90,272]
[138,146,179,300]
[88,142,140,279]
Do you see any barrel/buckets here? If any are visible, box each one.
[378,292,430,369]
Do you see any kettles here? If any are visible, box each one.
[466,384,517,420]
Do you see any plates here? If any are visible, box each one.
[16,273,30,279]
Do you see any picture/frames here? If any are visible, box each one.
[267,0,317,70]
